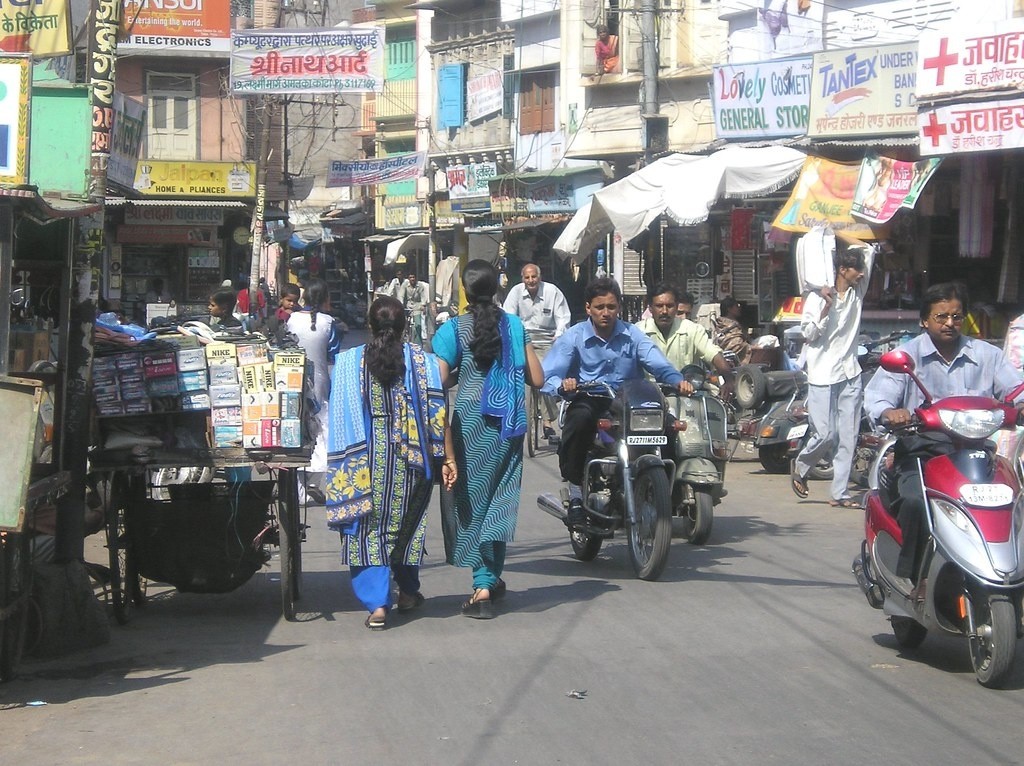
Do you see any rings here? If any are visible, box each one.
[728,391,736,398]
[565,381,570,383]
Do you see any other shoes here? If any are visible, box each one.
[543,426,555,438]
[568,498,586,522]
[307,487,327,505]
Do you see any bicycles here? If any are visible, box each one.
[525,337,554,458]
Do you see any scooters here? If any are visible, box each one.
[854,351,1023,687]
[655,363,738,541]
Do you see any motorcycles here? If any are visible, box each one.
[536,385,695,582]
[737,329,914,486]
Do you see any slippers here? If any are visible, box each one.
[398,593,424,611]
[833,500,861,508]
[791,459,808,498]
[364,614,384,630]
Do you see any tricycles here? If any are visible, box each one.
[87,311,317,624]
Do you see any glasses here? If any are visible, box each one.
[929,312,967,326]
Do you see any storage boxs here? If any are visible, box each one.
[93,335,305,448]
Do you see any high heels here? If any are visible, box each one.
[461,588,494,618]
[491,580,506,598]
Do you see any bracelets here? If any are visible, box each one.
[442,458,456,464]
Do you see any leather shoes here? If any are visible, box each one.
[910,579,928,601]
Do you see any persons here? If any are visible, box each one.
[142,278,172,325]
[632,280,737,404]
[372,268,427,348]
[794,230,875,506]
[208,286,244,335]
[715,298,780,371]
[503,264,571,439]
[536,278,694,533]
[590,25,619,81]
[431,260,544,608]
[863,277,1024,599]
[327,296,457,622]
[28,444,110,584]
[273,277,341,508]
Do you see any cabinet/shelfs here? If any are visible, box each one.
[323,268,343,309]
[756,252,780,325]
[122,245,184,321]
